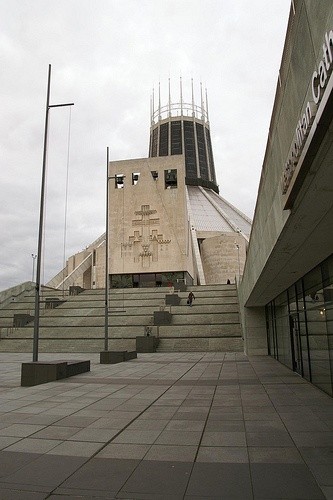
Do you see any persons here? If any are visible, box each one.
[187,291,195,308]
[226,279,231,286]
